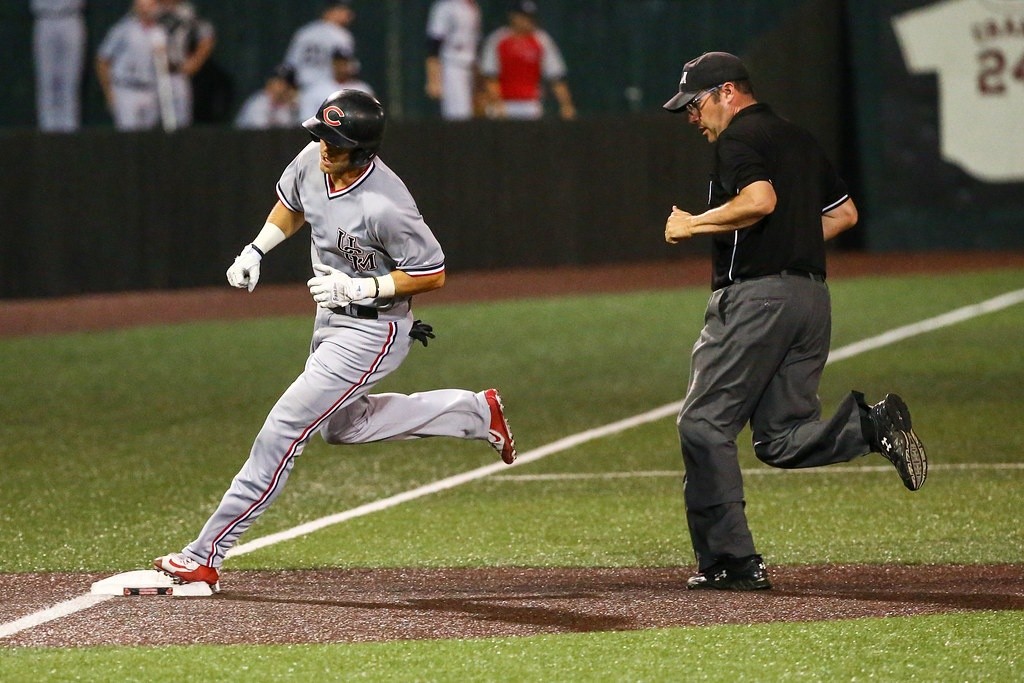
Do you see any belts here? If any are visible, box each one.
[328,295,413,320]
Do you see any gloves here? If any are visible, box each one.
[226,221,286,293]
[307,262,396,308]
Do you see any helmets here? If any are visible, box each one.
[303,88,387,167]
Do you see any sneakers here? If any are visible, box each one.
[152,549,221,588]
[868,392,928,491]
[484,387,516,463]
[687,555,773,592]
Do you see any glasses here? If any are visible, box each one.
[685,84,723,115]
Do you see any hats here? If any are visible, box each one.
[507,1,541,21]
[663,50,751,114]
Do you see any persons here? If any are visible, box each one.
[663,52,928,589]
[152,88,517,587]
[96,1,576,135]
